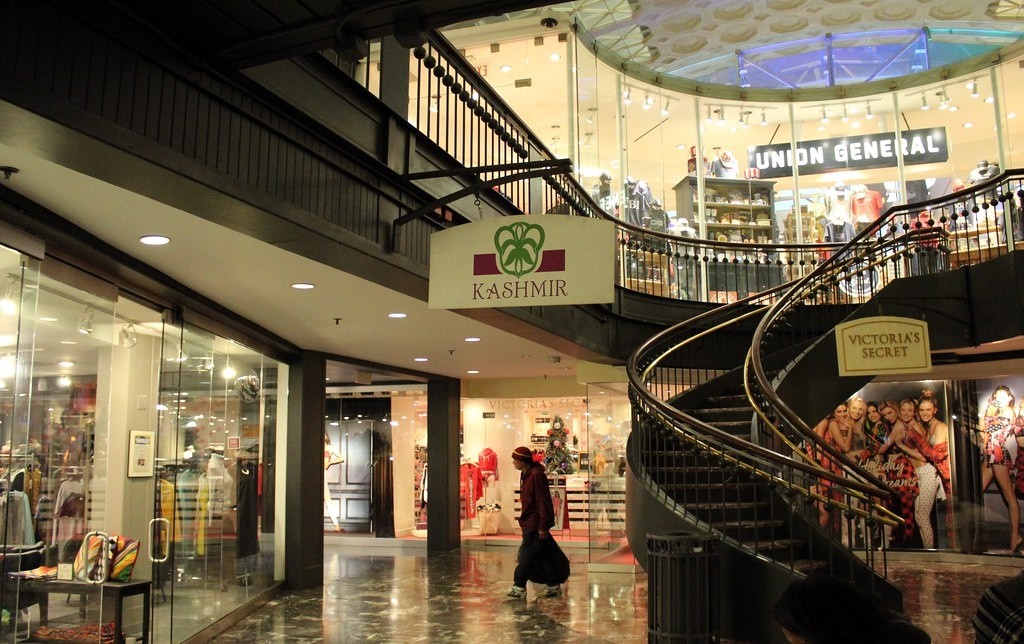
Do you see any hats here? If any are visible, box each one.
[511,446,533,462]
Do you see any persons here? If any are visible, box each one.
[806,389,952,549]
[910,211,938,273]
[969,159,1000,199]
[227,447,259,584]
[507,447,570,598]
[207,448,237,527]
[996,198,1022,243]
[981,386,1024,552]
[592,173,700,300]
[824,183,882,229]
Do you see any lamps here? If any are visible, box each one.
[661,98,670,115]
[820,106,832,122]
[624,87,632,104]
[742,111,752,128]
[842,106,849,122]
[175,342,188,362]
[937,91,949,110]
[77,305,95,336]
[642,92,650,110]
[0,279,22,316]
[705,106,713,123]
[738,107,745,125]
[760,108,768,126]
[120,322,139,351]
[921,92,930,112]
[865,101,874,119]
[37,377,48,392]
[942,86,952,103]
[714,106,726,127]
[971,79,980,98]
[586,110,596,122]
[584,133,592,148]
[203,352,214,370]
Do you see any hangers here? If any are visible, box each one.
[70,474,80,483]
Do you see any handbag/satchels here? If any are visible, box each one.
[523,532,571,584]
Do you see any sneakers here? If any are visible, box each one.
[536,585,563,598]
[507,586,527,598]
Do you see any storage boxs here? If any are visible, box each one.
[758,221,770,226]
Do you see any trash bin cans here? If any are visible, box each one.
[646,531,722,644]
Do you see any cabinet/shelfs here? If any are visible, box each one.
[618,247,669,297]
[673,176,787,302]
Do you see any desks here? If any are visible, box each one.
[941,240,1024,270]
[8,580,154,644]
